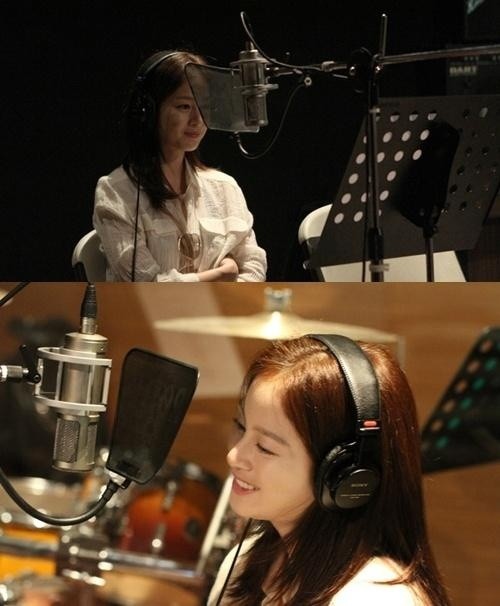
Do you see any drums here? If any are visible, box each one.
[71,448,243,606]
[0,476,83,584]
[0,571,124,606]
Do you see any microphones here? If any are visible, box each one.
[50,332,110,475]
[238,50,270,126]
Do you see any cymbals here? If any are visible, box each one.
[152,311,401,344]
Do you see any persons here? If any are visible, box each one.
[89,47,269,284]
[203,332,452,606]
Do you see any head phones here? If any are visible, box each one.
[123,49,189,134]
[312,332,385,512]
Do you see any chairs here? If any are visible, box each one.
[72,229,106,282]
[297,202,467,282]
